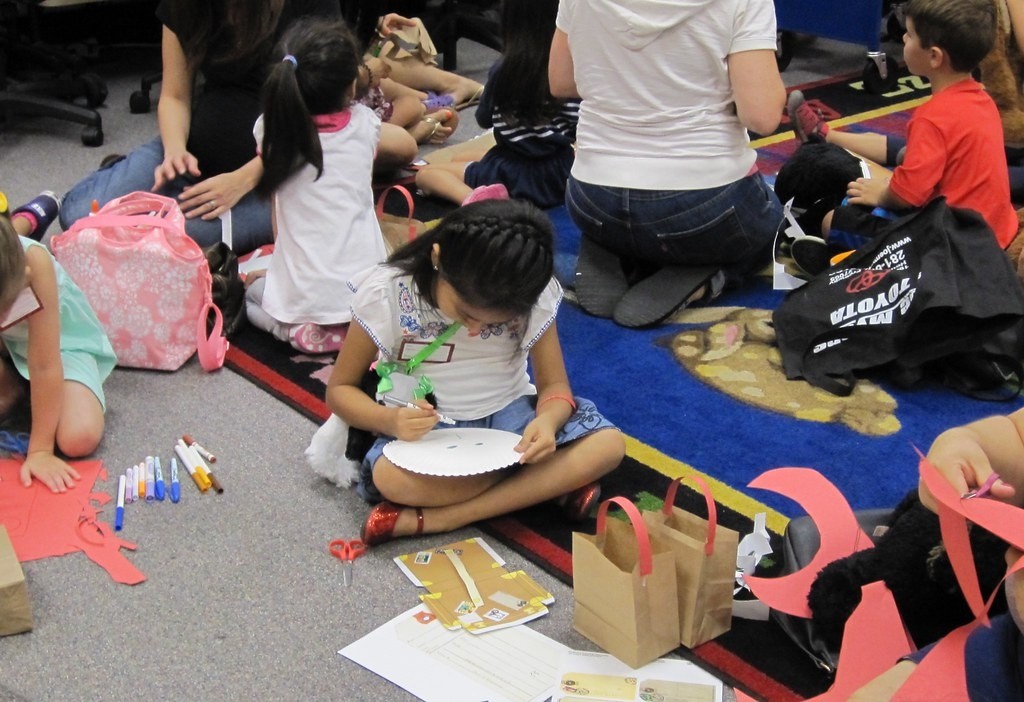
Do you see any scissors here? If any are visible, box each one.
[960,472,1001,500]
[328,538,367,588]
[372,33,390,61]
[0,429,30,461]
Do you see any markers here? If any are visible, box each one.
[383,394,457,426]
[113,434,224,532]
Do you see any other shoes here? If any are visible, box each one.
[460,184,509,206]
[575,233,631,318]
[550,483,601,524]
[360,503,424,546]
[614,261,721,329]
[421,89,453,115]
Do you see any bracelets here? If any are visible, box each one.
[536,392,576,415]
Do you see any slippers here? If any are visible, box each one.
[454,85,485,112]
[421,106,458,142]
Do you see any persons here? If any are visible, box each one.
[844,407,1024,702]
[357,12,485,146]
[787,0,1024,279]
[59,0,385,257]
[548,0,787,329]
[415,0,583,210]
[0,214,119,494]
[243,15,389,354]
[9,190,62,243]
[325,196,626,547]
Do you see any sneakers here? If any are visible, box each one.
[791,236,836,278]
[288,323,347,354]
[788,90,821,148]
[11,191,61,242]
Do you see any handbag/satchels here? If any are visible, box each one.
[572,475,739,669]
[771,204,1024,403]
[50,191,246,372]
[374,185,426,257]
[769,509,898,678]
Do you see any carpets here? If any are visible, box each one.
[223,60,1024,702]
